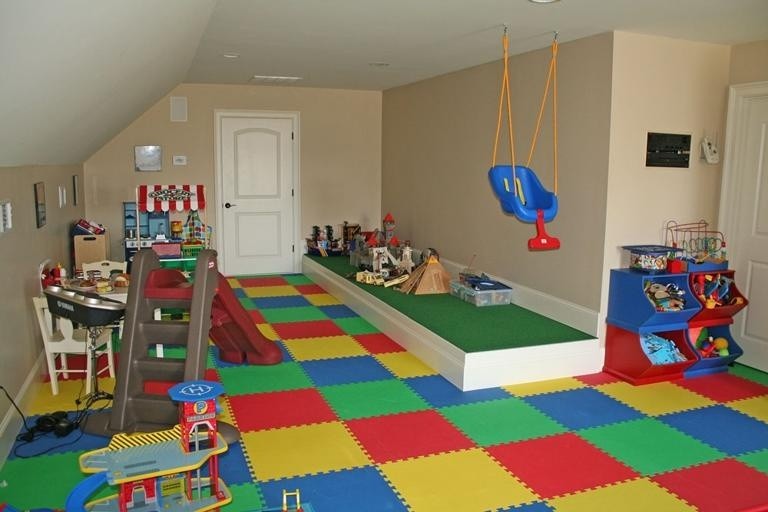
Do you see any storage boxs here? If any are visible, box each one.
[449,280,465,300]
[464,288,511,304]
[74,227,111,271]
[151,243,181,259]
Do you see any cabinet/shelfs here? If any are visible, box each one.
[602,270,750,386]
[124,201,170,274]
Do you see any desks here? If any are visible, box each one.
[42,278,164,358]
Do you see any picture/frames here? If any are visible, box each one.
[170,96,188,121]
[134,145,163,172]
[34,182,47,228]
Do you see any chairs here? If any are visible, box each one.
[81,259,127,340]
[32,295,114,396]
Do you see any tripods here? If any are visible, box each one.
[72,330,115,428]
